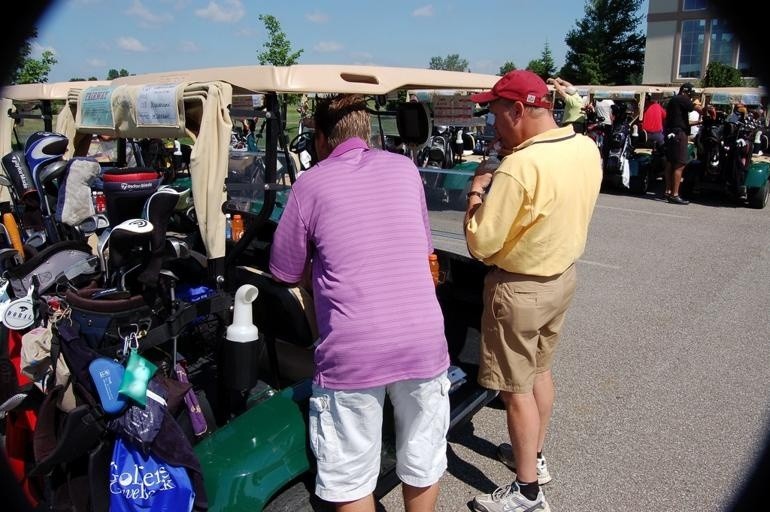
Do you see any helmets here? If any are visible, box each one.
[24,127,71,182]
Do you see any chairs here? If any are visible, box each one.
[160,230,321,386]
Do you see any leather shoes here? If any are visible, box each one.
[664,193,690,205]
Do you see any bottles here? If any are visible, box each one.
[482,151,501,199]
[225,213,245,242]
[428,253,440,289]
[91,190,107,214]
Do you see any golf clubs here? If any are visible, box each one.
[0,129,192,300]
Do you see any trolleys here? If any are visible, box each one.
[699,105,766,176]
[415,132,454,211]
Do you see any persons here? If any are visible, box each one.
[466,70,603,512]
[267,94,451,512]
[663,83,707,205]
[640,95,765,151]
[545,77,619,156]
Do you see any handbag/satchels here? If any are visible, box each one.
[29,396,209,511]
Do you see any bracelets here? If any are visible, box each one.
[465,191,482,202]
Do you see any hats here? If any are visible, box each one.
[680,81,701,98]
[467,69,556,110]
[565,86,578,95]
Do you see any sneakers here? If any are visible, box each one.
[496,441,554,486]
[471,482,550,512]
